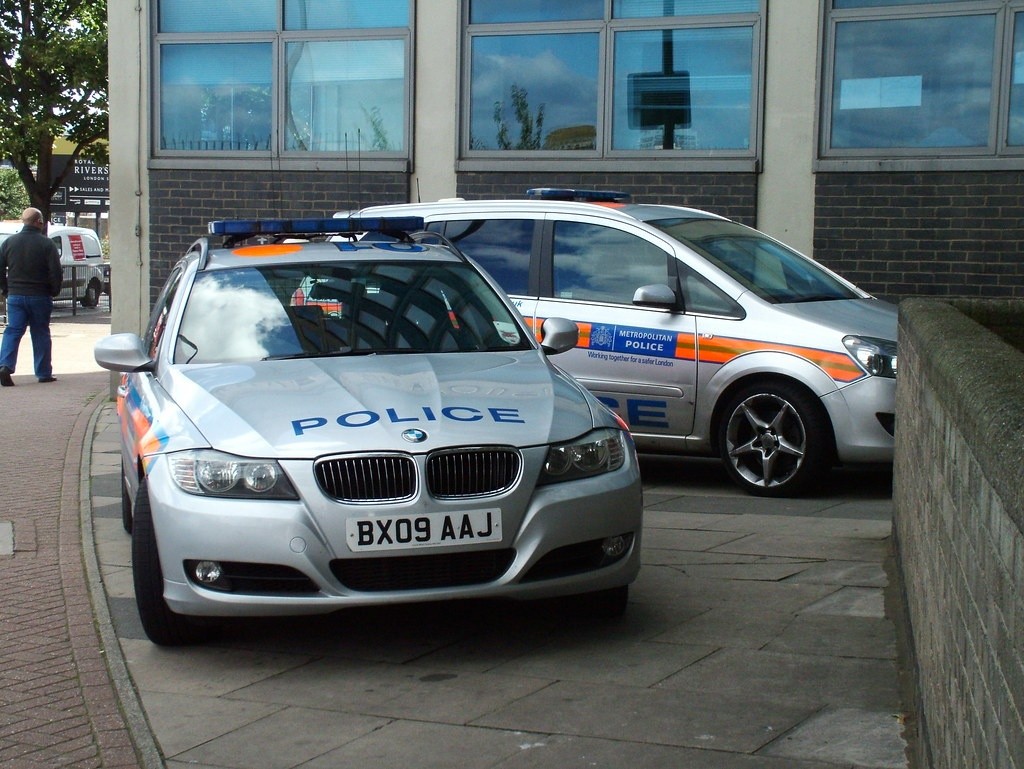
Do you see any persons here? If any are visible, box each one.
[0,207,63,386]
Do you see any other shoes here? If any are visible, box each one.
[39,377,57,382]
[0,367,15,386]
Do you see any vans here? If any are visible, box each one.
[0,219,104,309]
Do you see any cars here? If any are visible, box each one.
[101,261,110,295]
[324,188,898,498]
[94,219,643,645]
